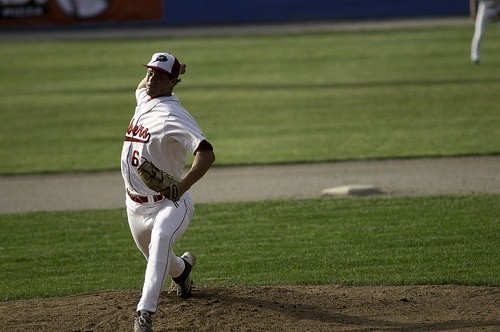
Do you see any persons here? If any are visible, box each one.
[120,52,216,332]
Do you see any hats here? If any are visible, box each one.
[141,52,180,78]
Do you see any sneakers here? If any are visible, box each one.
[134,310,153,332]
[168,252,196,296]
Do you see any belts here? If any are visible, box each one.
[127,188,163,204]
